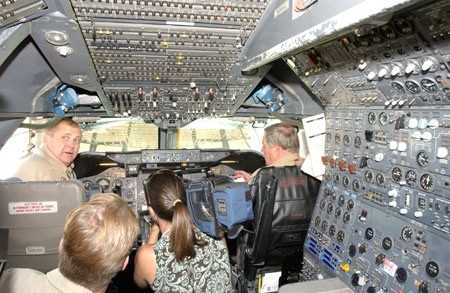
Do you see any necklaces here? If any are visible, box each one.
[161,228,171,238]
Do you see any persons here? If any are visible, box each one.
[134,171,233,293]
[14,118,80,181]
[0,193,139,293]
[234,124,320,273]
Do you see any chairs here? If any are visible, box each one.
[232,166,323,281]
[0,178,86,274]
